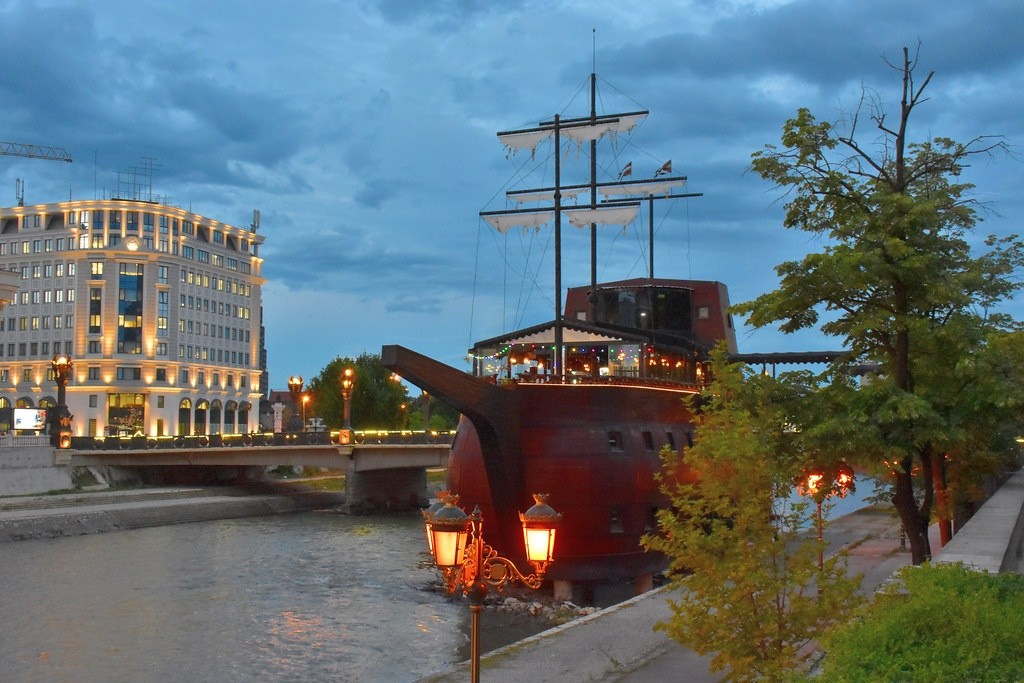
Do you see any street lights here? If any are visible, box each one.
[883,459,922,552]
[338,367,356,445]
[797,464,853,617]
[420,489,562,683]
[288,376,305,431]
[47,354,74,449]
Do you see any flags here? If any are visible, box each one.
[653,160,672,178]
[618,162,631,176]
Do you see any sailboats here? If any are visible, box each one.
[379,26,772,606]
